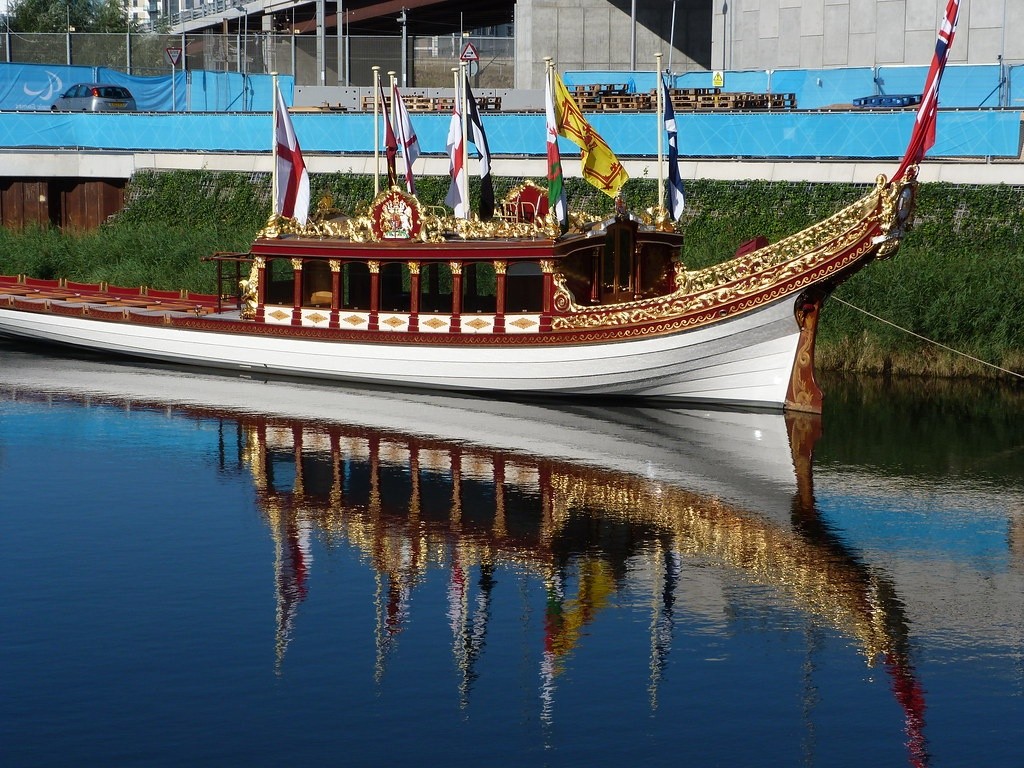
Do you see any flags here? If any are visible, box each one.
[464,75,494,222]
[442,105,464,218]
[276,89,309,224]
[553,71,630,198]
[545,74,566,225]
[891,0,961,183]
[660,73,685,222]
[377,78,420,195]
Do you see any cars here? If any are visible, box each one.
[50,82,138,114]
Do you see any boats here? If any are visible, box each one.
[0,154,920,417]
[1,340,929,768]
